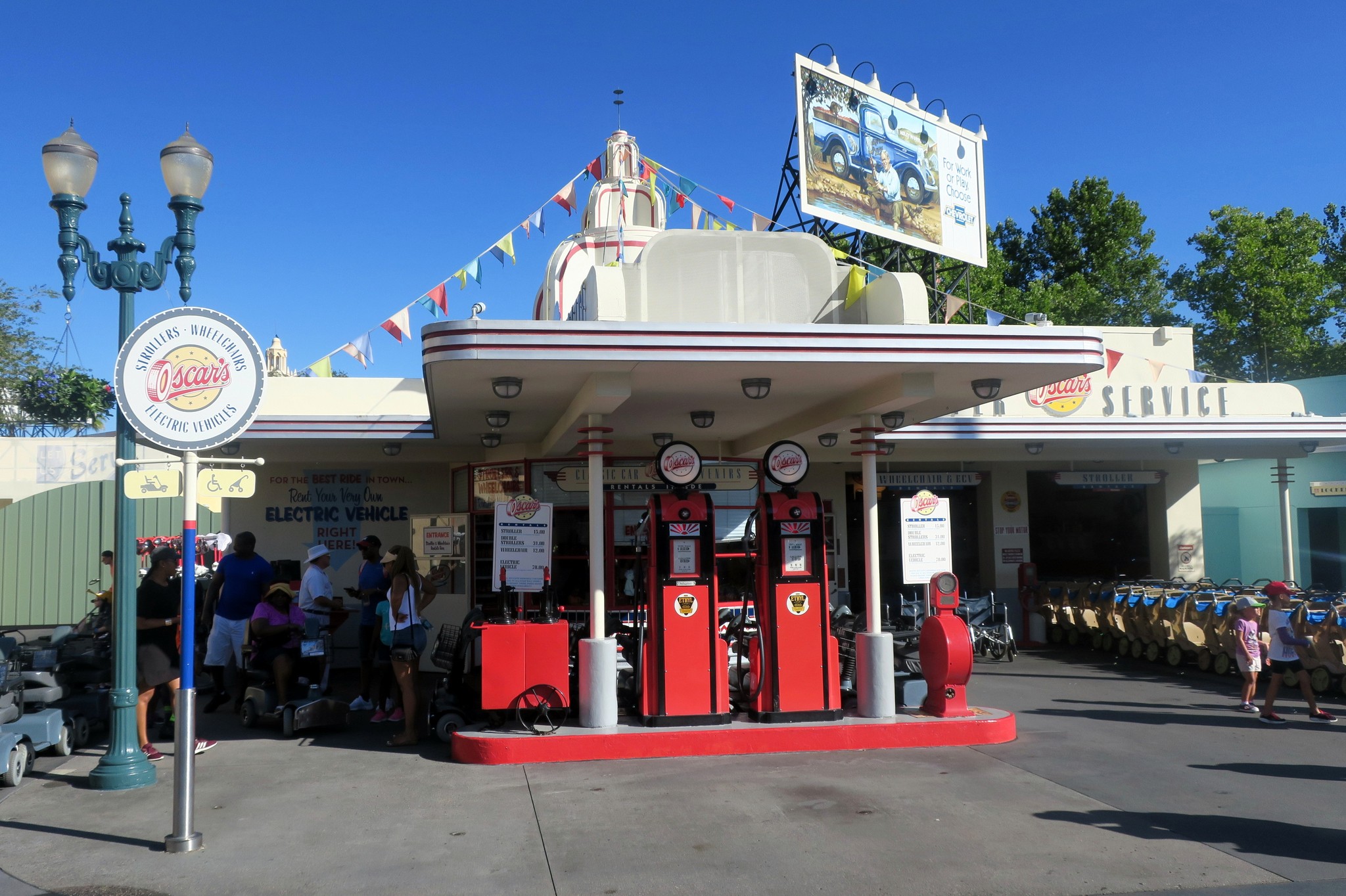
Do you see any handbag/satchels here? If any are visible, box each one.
[390,643,417,663]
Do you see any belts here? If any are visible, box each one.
[302,608,331,616]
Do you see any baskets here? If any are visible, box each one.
[430,624,462,670]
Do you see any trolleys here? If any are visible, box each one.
[1040,576,1346,693]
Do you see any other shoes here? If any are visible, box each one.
[204,690,232,714]
[1239,702,1260,713]
[385,729,418,746]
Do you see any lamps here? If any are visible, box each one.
[485,410,511,428]
[971,379,1001,399]
[652,433,673,447]
[890,82,920,112]
[808,43,840,74]
[851,61,881,91]
[881,411,905,429]
[959,114,988,141]
[1025,443,1044,455]
[876,442,896,456]
[490,376,523,399]
[741,378,772,400]
[1300,441,1319,453]
[925,99,950,124]
[818,433,839,447]
[480,433,501,448]
[690,411,715,428]
[1164,442,1183,454]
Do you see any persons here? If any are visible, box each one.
[90,529,438,757]
[1260,581,1337,724]
[1227,597,1269,713]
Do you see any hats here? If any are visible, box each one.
[263,583,296,599]
[150,546,182,562]
[1236,596,1266,610]
[1264,581,1296,595]
[303,544,334,565]
[356,535,382,548]
[380,551,398,563]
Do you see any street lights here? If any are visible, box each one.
[41,117,214,789]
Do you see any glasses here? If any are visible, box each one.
[358,546,370,552]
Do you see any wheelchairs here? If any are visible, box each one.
[955,591,1018,662]
[898,593,936,630]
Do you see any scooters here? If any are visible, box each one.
[0,626,109,786]
[240,616,350,737]
[428,601,648,743]
[718,604,920,713]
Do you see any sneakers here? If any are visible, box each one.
[1309,709,1338,723]
[387,708,405,721]
[1259,709,1287,724]
[350,696,375,711]
[385,697,394,711]
[370,710,388,722]
[141,742,164,761]
[194,736,218,754]
